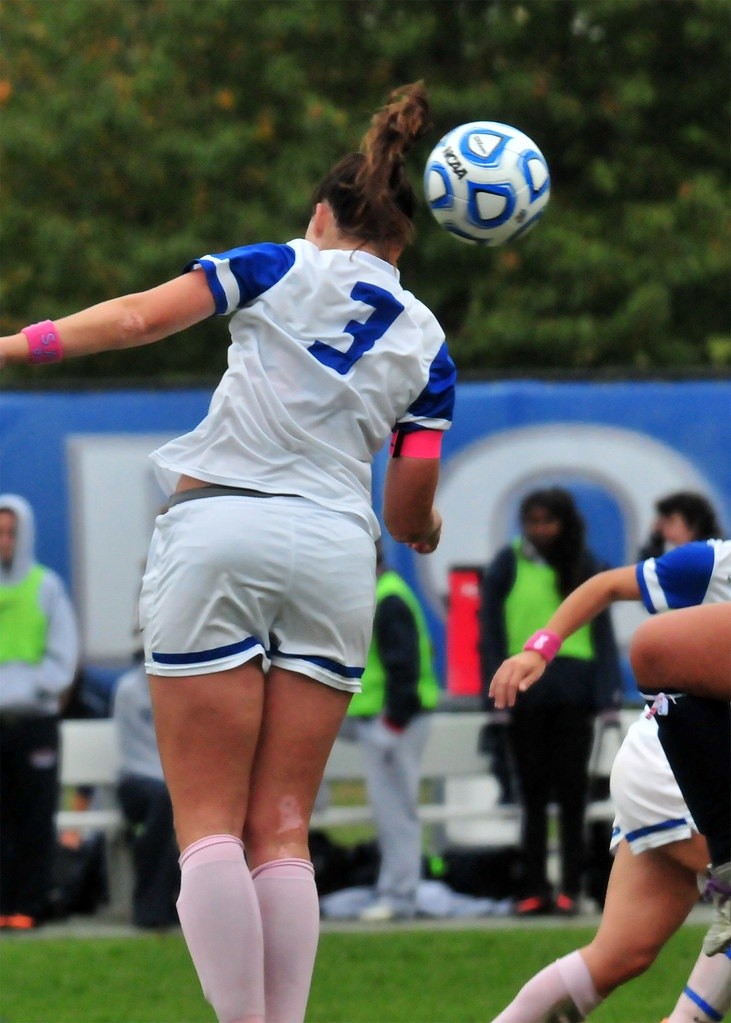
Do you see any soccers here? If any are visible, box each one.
[422,118,552,251]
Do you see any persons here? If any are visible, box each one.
[0,75,460,1022]
[0,487,83,928]
[476,480,731,1022]
[108,641,200,933]
[355,537,444,922]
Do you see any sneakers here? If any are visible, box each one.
[0,898,44,929]
[359,894,417,923]
[514,889,582,920]
